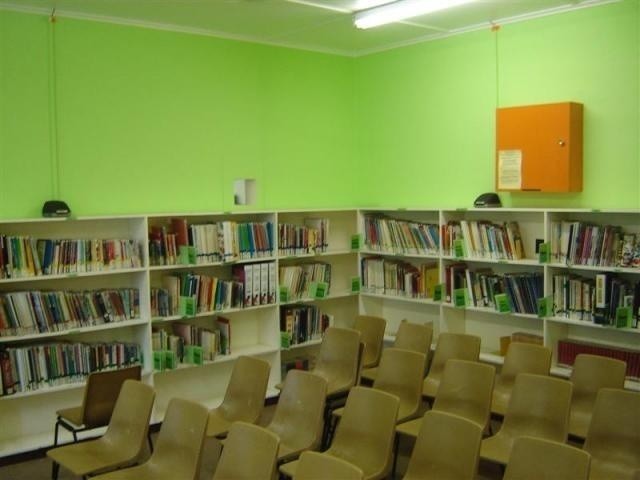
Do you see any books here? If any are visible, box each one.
[362,213,439,298]
[499,332,544,357]
[0,235,144,399]
[279,218,331,371]
[150,219,276,371]
[441,221,543,314]
[551,220,640,328]
[558,338,640,381]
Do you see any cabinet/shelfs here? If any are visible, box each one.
[146,213,279,427]
[495,101,584,194]
[440,210,545,364]
[276,210,360,387]
[357,209,440,350]
[545,212,640,388]
[0,217,148,459]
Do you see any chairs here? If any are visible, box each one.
[275,328,366,448]
[352,315,385,369]
[206,355,271,437]
[395,359,496,476]
[420,332,481,395]
[46,379,156,480]
[292,450,363,479]
[361,322,432,384]
[279,386,401,479]
[501,437,590,480]
[567,353,627,440]
[402,411,483,480]
[479,374,573,466]
[211,422,279,480]
[582,388,640,480]
[86,394,209,480]
[54,366,153,455]
[490,342,552,416]
[221,370,328,461]
[329,348,425,446]
